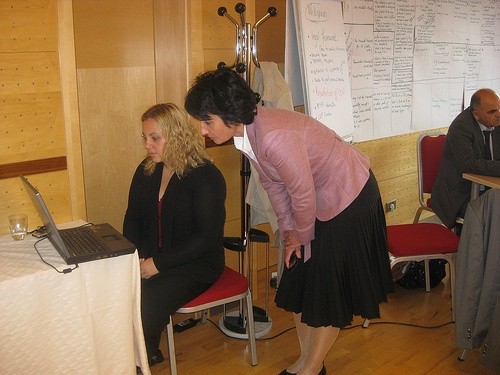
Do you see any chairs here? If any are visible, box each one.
[414,132,464,233]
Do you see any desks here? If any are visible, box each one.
[463,174,500,201]
[0,220,151,375]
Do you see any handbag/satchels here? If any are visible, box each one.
[396,259,447,290]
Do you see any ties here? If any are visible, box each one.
[484,130,493,189]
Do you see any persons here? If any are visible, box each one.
[183,69,394,375]
[431,89,500,230]
[123,103,215,373]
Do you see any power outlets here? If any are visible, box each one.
[386,199,396,214]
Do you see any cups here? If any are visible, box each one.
[7,214,28,240]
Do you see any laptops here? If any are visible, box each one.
[20,175,137,266]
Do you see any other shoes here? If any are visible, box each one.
[279,368,296,375]
[138,349,164,373]
[318,360,326,375]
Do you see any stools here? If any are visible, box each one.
[386,222,460,323]
[165,266,257,375]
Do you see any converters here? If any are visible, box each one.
[173,318,197,333]
